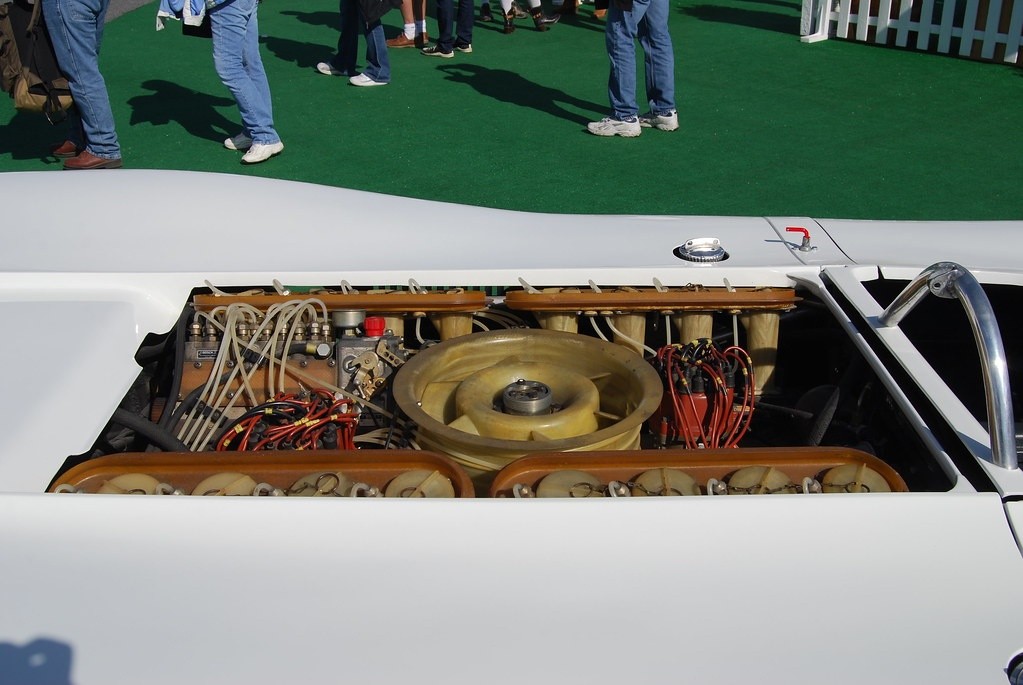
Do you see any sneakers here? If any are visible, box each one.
[240,141,284,164]
[639,109,679,131]
[588,116,642,138]
[316,62,356,75]
[385,31,422,48]
[421,40,454,57]
[420,32,429,45]
[349,73,388,86]
[451,39,472,52]
[224,132,253,150]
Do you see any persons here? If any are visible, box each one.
[587,0,679,138]
[209,0,286,165]
[420,0,474,58]
[478,0,610,35]
[0,0,124,171]
[317,0,393,88]
[385,0,430,48]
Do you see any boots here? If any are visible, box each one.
[529,5,562,32]
[501,7,515,34]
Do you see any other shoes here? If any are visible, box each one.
[63,149,122,169]
[592,8,607,19]
[583,0,593,4]
[479,3,494,21]
[48,139,86,156]
[502,1,528,18]
[552,0,579,16]
